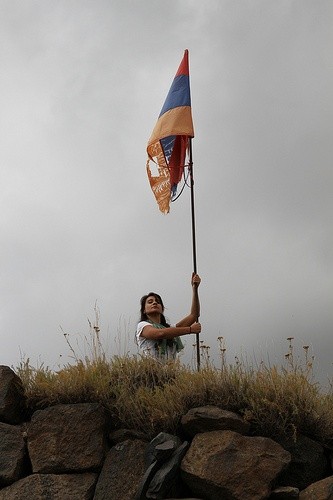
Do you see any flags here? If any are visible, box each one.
[146,51,194,213]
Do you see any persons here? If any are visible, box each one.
[136,273,201,367]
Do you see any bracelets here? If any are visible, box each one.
[189,326,193,334]
